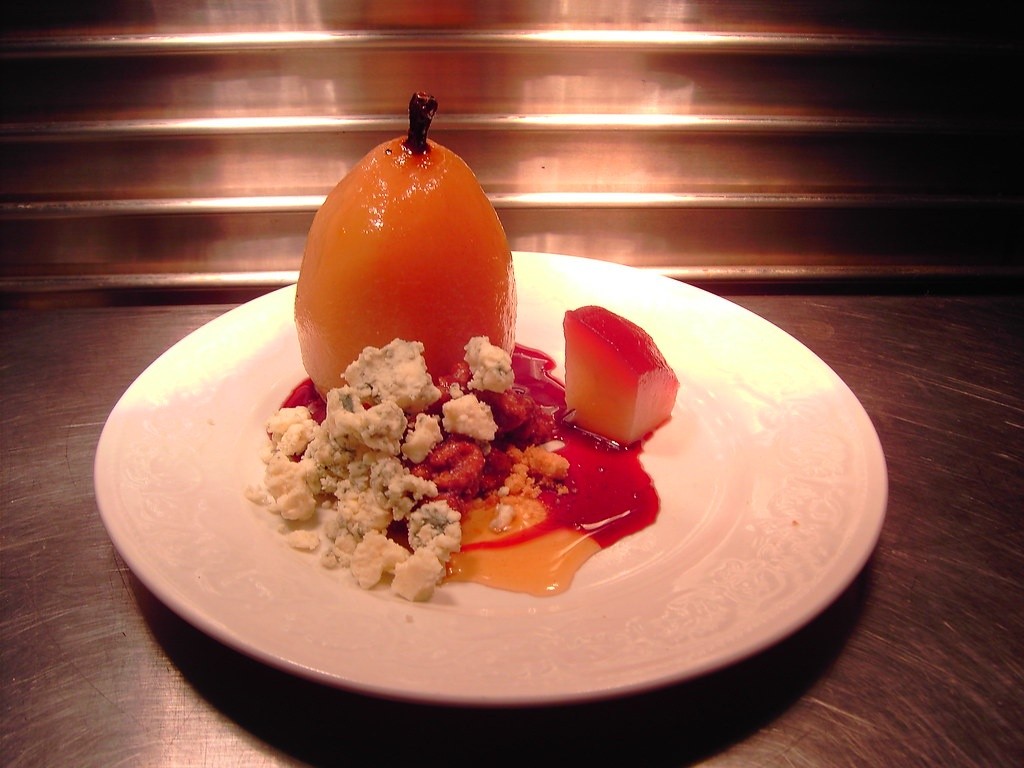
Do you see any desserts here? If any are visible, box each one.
[238,88,679,603]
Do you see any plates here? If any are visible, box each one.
[91,250,890,709]
